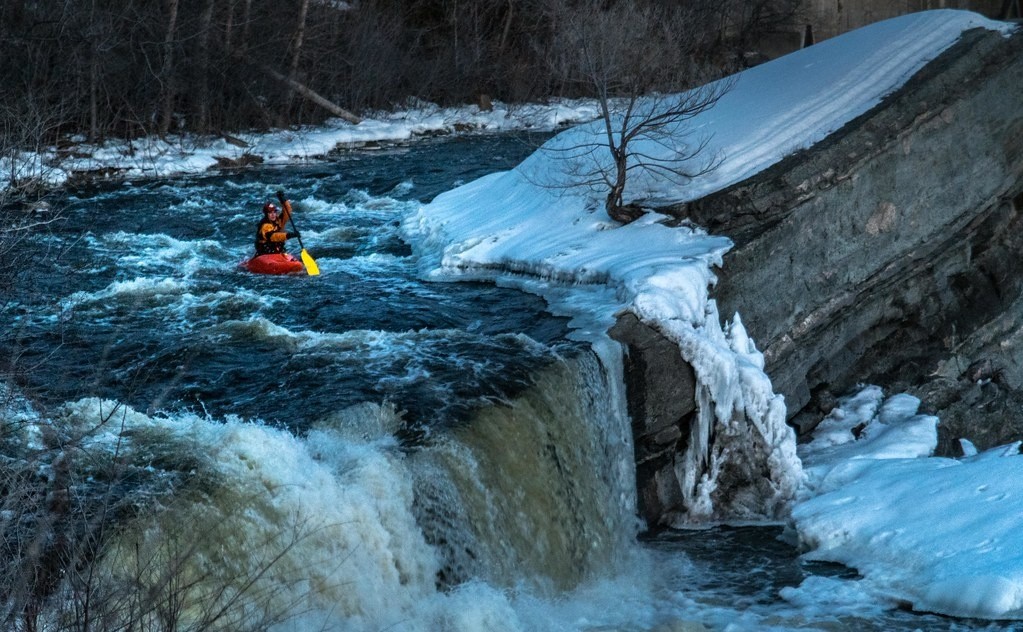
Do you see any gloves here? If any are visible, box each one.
[286,231,300,239]
[276,191,286,204]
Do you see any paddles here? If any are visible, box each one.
[275,190,320,276]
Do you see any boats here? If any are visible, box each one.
[237,253,303,275]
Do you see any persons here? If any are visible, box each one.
[255,190,302,254]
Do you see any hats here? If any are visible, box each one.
[263,204,277,212]
